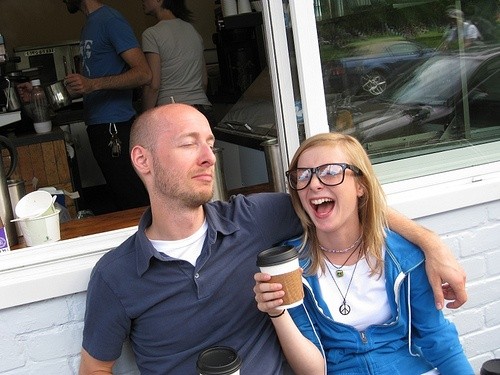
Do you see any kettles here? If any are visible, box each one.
[0,133,18,246]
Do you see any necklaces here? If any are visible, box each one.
[318,240,362,277]
[317,223,363,252]
[323,246,363,316]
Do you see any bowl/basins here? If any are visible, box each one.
[34,120,52,133]
[14,190,55,220]
[18,209,62,247]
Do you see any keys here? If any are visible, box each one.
[109,133,127,158]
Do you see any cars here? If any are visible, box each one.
[332,36,435,100]
[319,42,500,163]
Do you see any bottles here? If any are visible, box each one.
[28,79,49,123]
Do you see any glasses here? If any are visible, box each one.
[284,163,363,190]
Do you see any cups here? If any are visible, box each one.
[45,78,73,112]
[2,86,22,112]
[256,244,305,309]
[479,358,500,375]
[6,178,28,238]
[197,345,242,375]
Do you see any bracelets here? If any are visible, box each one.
[267,308,285,318]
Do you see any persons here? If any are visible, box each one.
[81,104,465,375]
[60,0,150,215]
[253,130,476,375]
[434,4,480,43]
[139,0,210,119]
